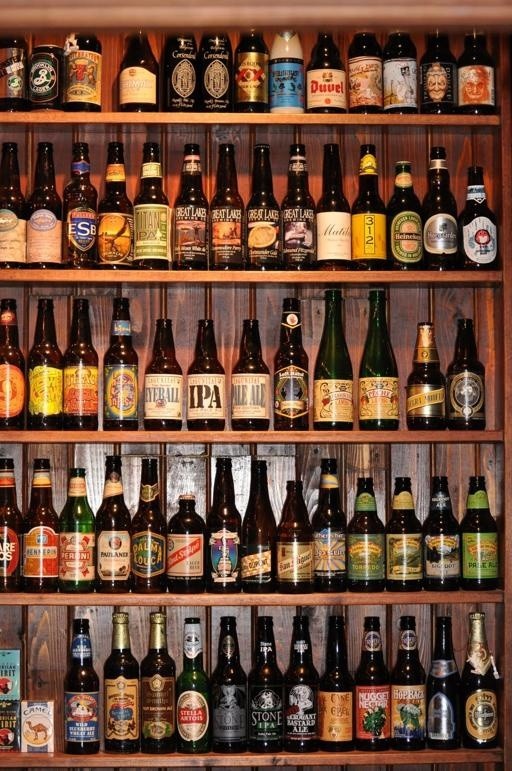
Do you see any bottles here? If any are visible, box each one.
[0,137,501,270]
[0,293,499,430]
[0,26,501,112]
[0,455,502,589]
[66,612,500,753]
